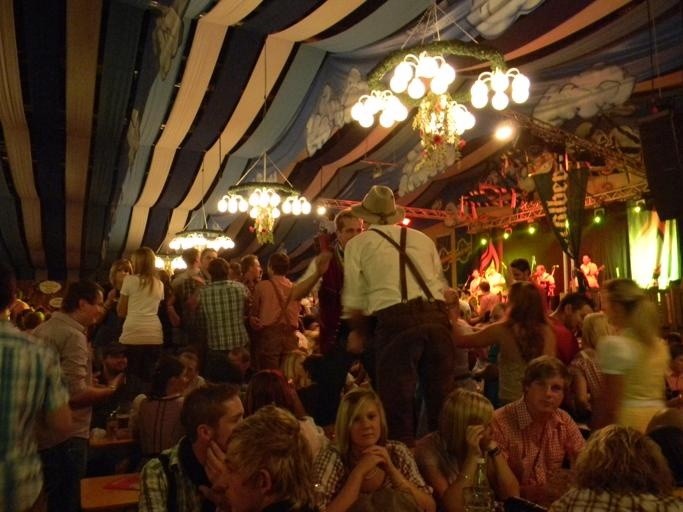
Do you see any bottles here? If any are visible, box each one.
[106,407,136,442]
[473,457,492,512]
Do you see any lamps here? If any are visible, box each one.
[345,2,532,147]
[479,195,643,246]
[212,33,326,220]
[163,150,237,253]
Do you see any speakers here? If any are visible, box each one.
[637,109,683,222]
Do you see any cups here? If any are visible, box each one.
[460,485,495,511]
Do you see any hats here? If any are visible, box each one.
[351,184,405,225]
